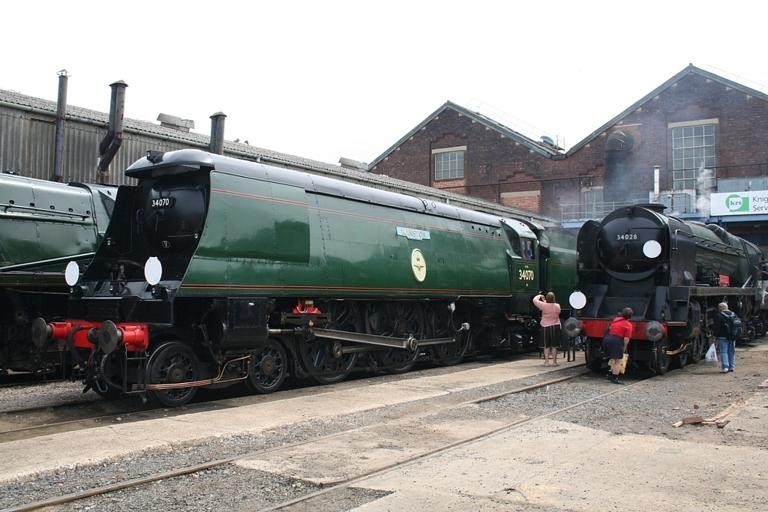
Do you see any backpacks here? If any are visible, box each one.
[720,311,744,340]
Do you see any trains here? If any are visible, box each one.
[568,201,768,376]
[2,168,120,383]
[32,147,578,405]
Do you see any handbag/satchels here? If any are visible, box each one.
[603,323,610,336]
[608,349,629,375]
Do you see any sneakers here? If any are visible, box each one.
[719,367,735,373]
[539,362,561,367]
[604,373,625,385]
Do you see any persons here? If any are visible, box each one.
[599,307,635,386]
[531,291,562,368]
[712,301,738,374]
